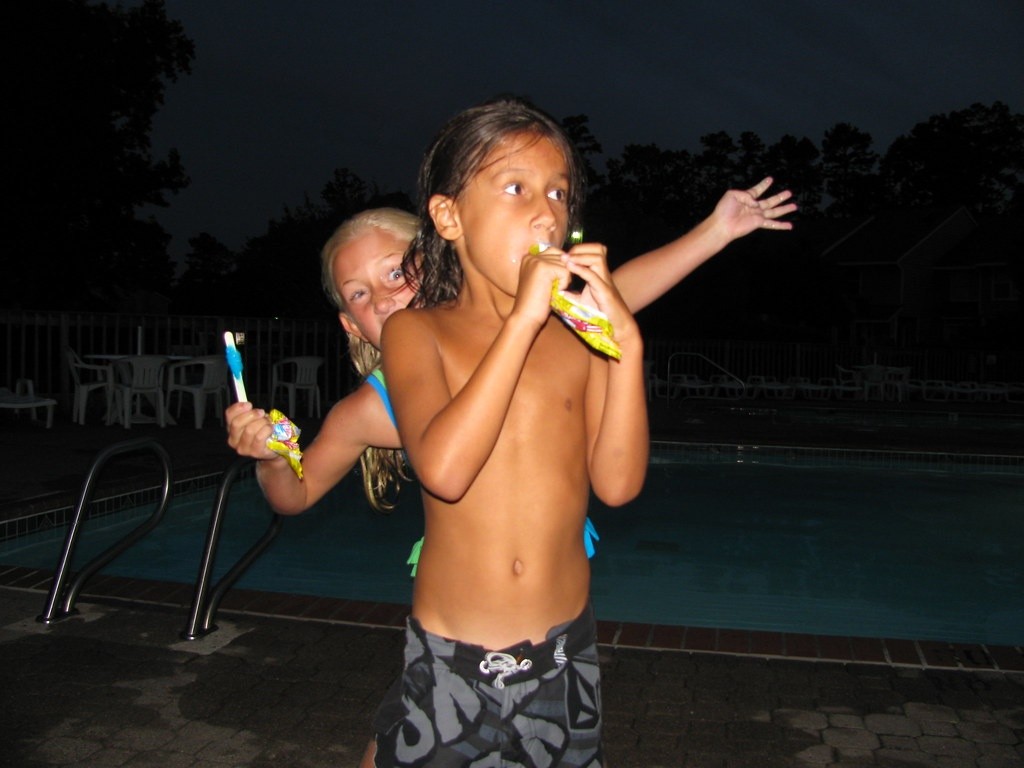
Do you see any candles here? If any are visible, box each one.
[138,326,142,356]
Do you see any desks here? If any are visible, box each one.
[852,366,905,401]
[83,354,192,423]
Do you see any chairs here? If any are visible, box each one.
[0,350,230,430]
[269,356,326,418]
[648,363,1024,404]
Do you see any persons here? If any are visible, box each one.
[224,176,797,768]
[380,101,648,768]
